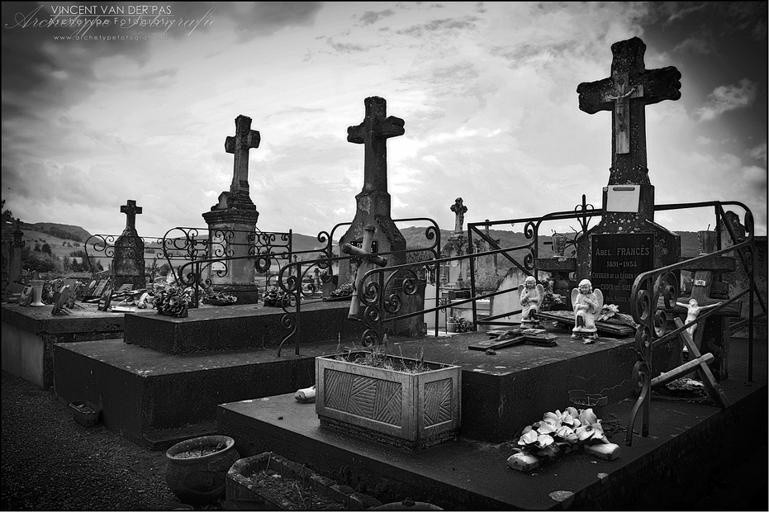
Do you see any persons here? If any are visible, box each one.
[605,84,638,132]
[574,278,597,330]
[519,275,540,320]
[674,297,723,352]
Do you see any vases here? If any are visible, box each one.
[315,350,463,459]
[166,434,241,507]
[224,451,382,510]
